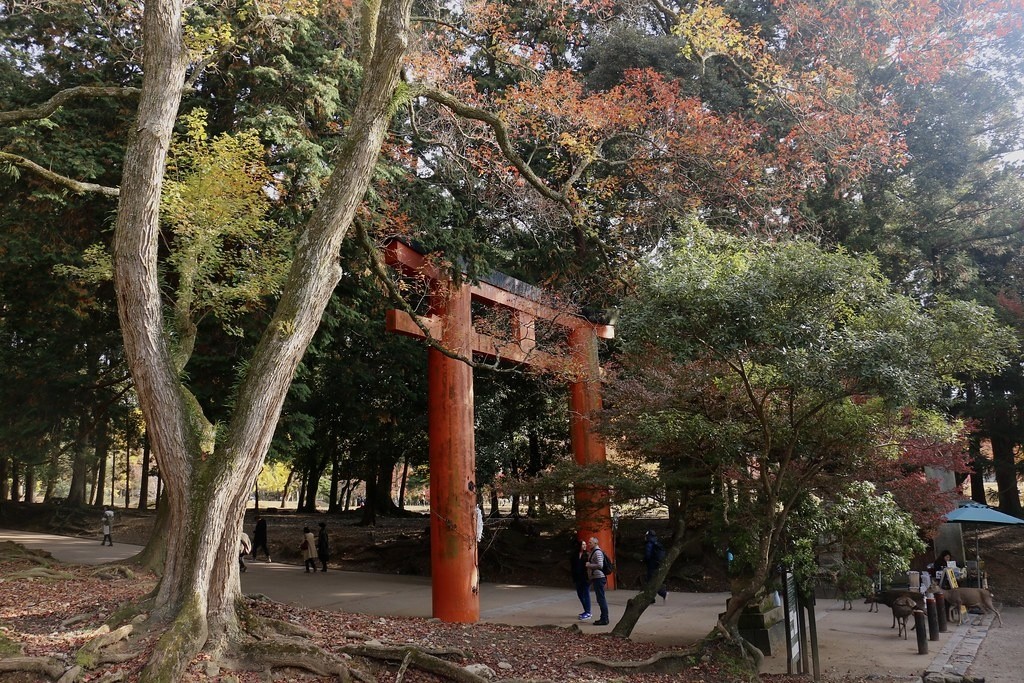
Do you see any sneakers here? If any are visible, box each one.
[578,613,592,620]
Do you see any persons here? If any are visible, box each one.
[297,525,319,573]
[316,521,331,573]
[239,526,252,572]
[351,500,364,513]
[933,549,952,571]
[100,504,114,547]
[420,490,426,507]
[570,539,594,621]
[641,529,670,606]
[251,514,272,563]
[584,535,614,626]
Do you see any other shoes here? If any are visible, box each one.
[243,567,246,573]
[650,599,656,604]
[108,544,113,546]
[593,620,609,625]
[266,559,271,562]
[664,592,669,601]
[321,567,327,572]
[249,560,256,562]
[100,543,105,545]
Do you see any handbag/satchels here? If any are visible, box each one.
[300,534,308,551]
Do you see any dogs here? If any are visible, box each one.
[925,580,1003,627]
[865,588,925,630]
[892,596,917,640]
[834,568,879,613]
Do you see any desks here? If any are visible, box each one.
[931,576,988,614]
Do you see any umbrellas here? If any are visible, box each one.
[940,500,1024,588]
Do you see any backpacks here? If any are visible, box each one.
[589,549,612,575]
[239,533,251,556]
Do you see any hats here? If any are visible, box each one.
[644,530,656,536]
[318,522,326,527]
